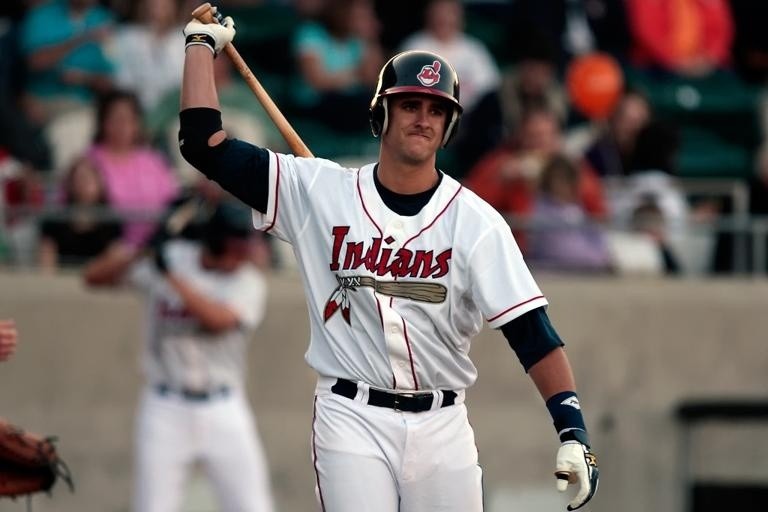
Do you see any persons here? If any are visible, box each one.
[0,319,17,361]
[454,52,722,272]
[3,3,291,273]
[178,6,599,512]
[289,2,392,138]
[84,182,276,512]
[397,3,507,114]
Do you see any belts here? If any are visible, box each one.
[155,382,233,406]
[332,378,454,412]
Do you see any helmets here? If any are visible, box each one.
[368,50,464,150]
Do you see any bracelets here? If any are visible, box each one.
[546,391,588,434]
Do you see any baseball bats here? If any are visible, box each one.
[191,3,313,157]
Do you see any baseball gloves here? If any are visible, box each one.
[0,422,74,507]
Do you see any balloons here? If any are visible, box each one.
[632,1,734,75]
[566,53,624,123]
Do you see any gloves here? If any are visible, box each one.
[183,6,236,58]
[554,427,598,511]
